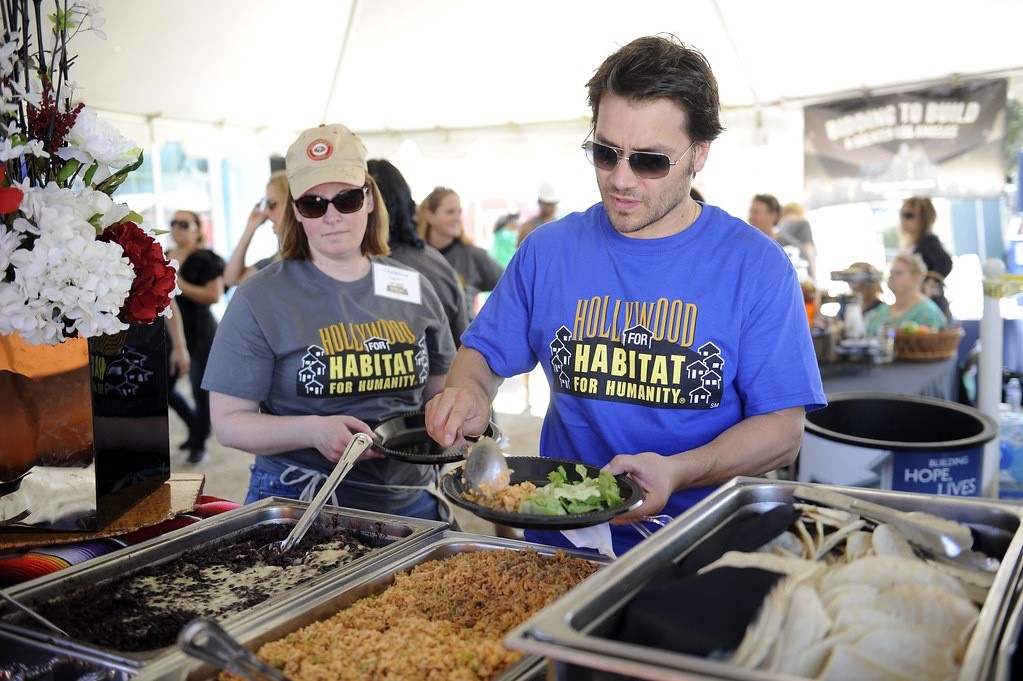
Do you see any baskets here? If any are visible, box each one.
[881,321,965,359]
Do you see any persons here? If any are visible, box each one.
[747,195,953,332]
[425,36,827,558]
[201,124,477,521]
[367,159,560,408]
[164,171,288,463]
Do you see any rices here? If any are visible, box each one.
[449,436,537,513]
[218,544,599,681]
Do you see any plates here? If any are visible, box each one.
[369,409,500,464]
[442,457,642,528]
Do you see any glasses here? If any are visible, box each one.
[898,211,919,218]
[170,220,188,229]
[580,126,696,180]
[294,182,367,218]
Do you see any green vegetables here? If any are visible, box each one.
[518,463,625,516]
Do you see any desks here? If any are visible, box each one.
[821,355,958,401]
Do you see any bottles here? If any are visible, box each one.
[1005,377,1021,414]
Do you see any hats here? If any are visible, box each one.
[537,182,562,203]
[283,122,367,201]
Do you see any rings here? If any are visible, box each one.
[428,432,435,437]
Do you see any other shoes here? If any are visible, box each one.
[179,432,206,462]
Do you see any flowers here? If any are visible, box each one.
[0,0,181,346]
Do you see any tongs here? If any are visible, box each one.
[793,484,1001,589]
